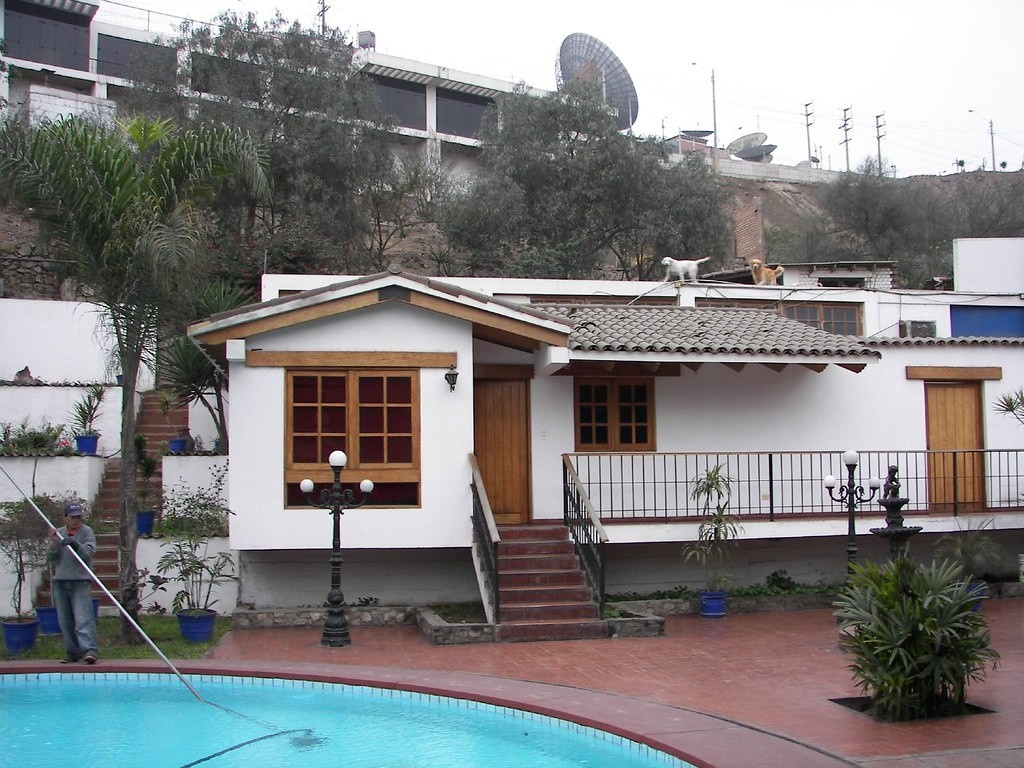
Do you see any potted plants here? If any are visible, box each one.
[133,432,160,535]
[682,461,747,619]
[0,489,100,657]
[156,515,237,645]
[154,386,188,455]
[64,382,110,456]
[931,515,999,613]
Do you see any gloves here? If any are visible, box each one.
[60,538,79,551]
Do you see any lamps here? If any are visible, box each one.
[445,364,460,393]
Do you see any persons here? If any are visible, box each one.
[47,504,99,664]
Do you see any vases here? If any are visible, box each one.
[116,372,124,386]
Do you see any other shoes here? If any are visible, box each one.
[84,656,97,664]
[60,656,77,663]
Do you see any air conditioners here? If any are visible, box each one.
[898,319,938,338]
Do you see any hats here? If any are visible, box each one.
[64,504,83,517]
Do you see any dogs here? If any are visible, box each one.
[661,256,710,283]
[749,259,784,285]
